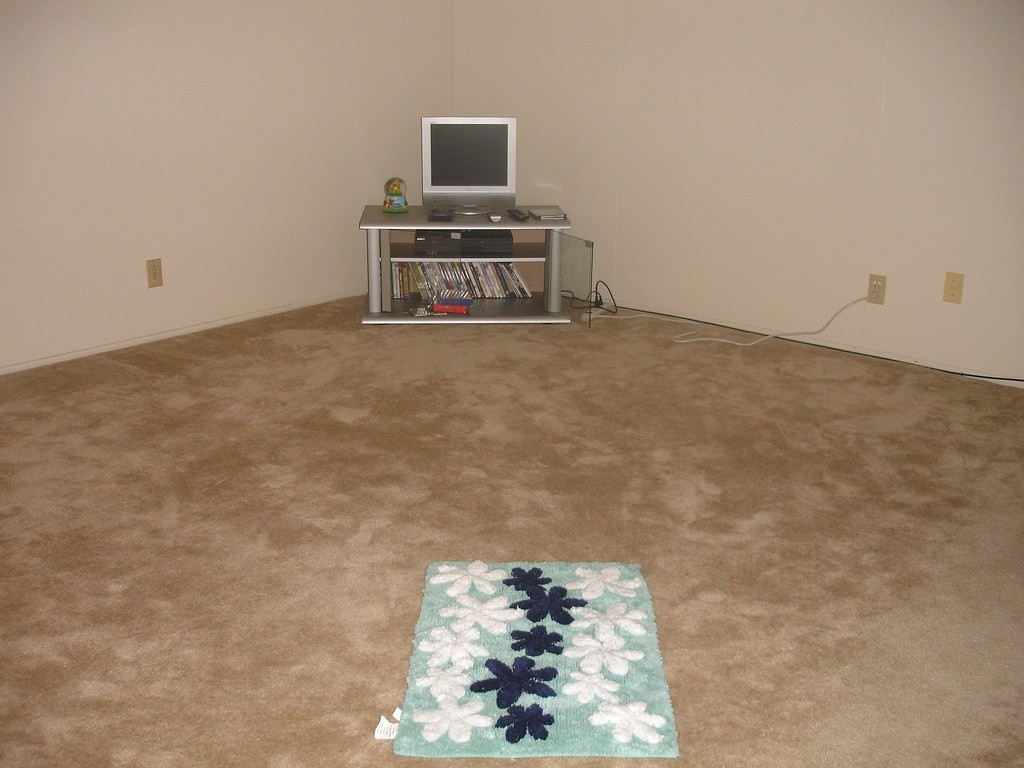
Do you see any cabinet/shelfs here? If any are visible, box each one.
[358,205,594,329]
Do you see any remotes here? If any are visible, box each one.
[487,212,501,222]
[507,209,529,220]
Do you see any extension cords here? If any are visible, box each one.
[580,304,610,323]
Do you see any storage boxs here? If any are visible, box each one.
[437,289,473,305]
[415,230,461,258]
[462,229,513,255]
[433,303,467,313]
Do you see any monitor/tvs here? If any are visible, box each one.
[422,117,516,215]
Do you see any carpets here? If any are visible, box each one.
[390,560,680,758]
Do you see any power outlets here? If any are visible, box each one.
[943,272,964,304]
[147,258,162,288]
[867,274,885,305]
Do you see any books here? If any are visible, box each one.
[529,208,567,221]
[391,262,532,314]
